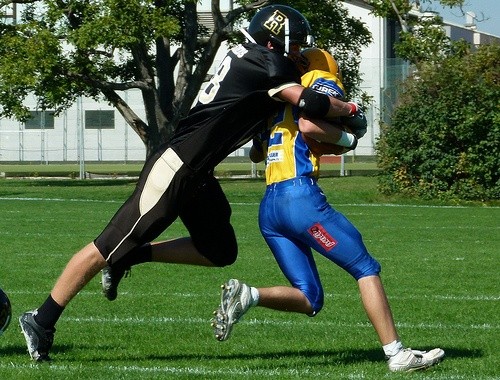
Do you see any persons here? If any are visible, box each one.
[18,6,367,359]
[210,48,445,373]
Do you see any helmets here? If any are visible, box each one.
[297,47,339,76]
[248,4,313,51]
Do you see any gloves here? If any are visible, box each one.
[346,103,368,138]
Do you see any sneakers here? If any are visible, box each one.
[18,308,56,364]
[100,265,132,301]
[385,345,445,373]
[210,278,254,343]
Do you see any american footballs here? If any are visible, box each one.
[301,123,353,155]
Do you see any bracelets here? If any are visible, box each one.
[336,131,354,147]
